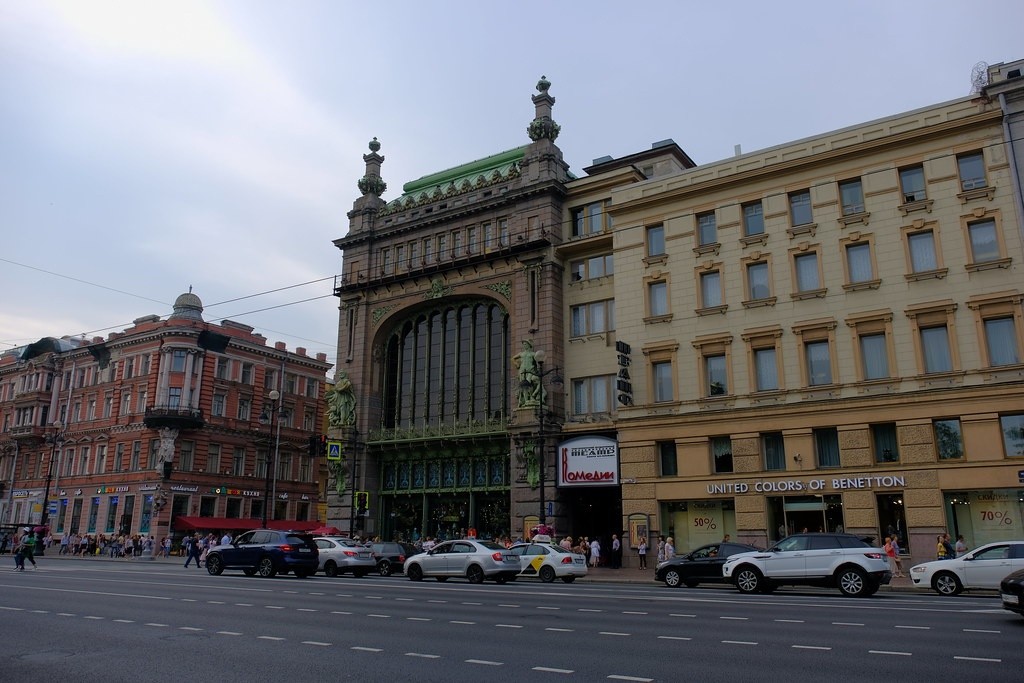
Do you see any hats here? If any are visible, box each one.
[23,527,30,532]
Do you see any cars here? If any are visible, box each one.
[313,535,378,579]
[999,567,1024,615]
[368,542,421,578]
[653,542,759,589]
[402,539,522,584]
[909,540,1024,596]
[508,535,589,584]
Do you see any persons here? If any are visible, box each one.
[779,525,792,539]
[709,552,718,556]
[494,529,538,548]
[59,532,171,559]
[657,536,674,560]
[460,526,476,540]
[638,538,647,569]
[611,535,620,569]
[723,534,729,542]
[335,372,352,424]
[159,428,175,460]
[356,536,381,544]
[885,536,906,577]
[512,340,540,401]
[179,530,233,568]
[550,536,606,567]
[937,534,967,560]
[414,536,441,552]
[0,527,53,572]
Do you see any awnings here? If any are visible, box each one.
[174,515,326,530]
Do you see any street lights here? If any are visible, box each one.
[816,494,827,533]
[342,420,370,538]
[520,348,565,536]
[259,389,292,528]
[32,420,66,556]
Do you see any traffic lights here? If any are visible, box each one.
[319,434,328,457]
[356,492,369,515]
[307,436,317,455]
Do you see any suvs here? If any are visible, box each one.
[721,533,893,598]
[204,528,320,578]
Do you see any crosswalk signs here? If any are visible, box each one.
[327,442,343,462]
